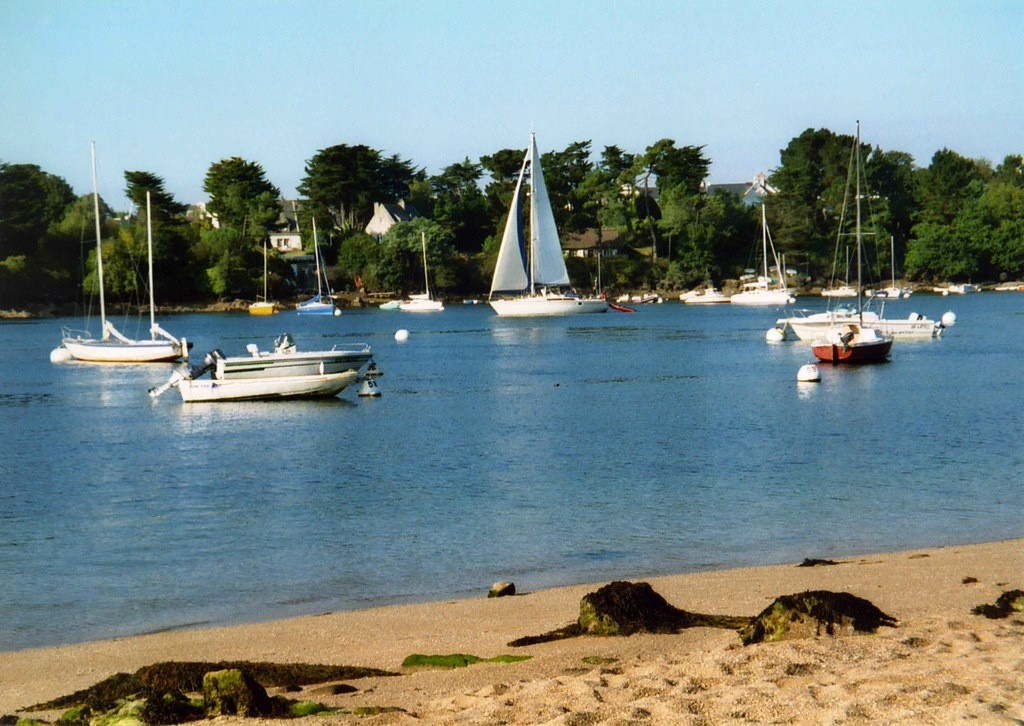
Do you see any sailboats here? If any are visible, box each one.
[50,140,194,366]
[379,234,446,314]
[248,237,288,315]
[487,132,610,319]
[616,120,1024,366]
[290,216,343,317]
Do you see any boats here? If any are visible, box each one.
[147,332,374,404]
